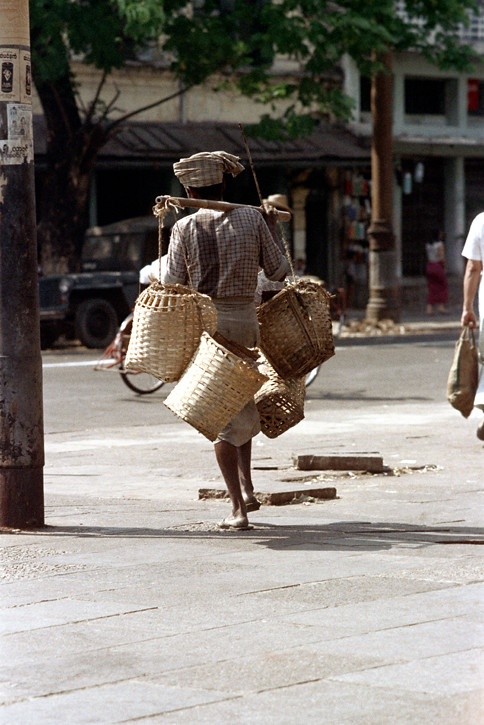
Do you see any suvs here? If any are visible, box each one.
[40,215,188,349]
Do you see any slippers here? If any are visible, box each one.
[216,518,253,530]
[244,502,263,512]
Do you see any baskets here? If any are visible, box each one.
[162,329,269,443]
[124,281,218,381]
[255,279,336,380]
[253,348,306,439]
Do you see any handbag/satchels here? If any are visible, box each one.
[446,324,479,418]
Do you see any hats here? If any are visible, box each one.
[263,193,293,214]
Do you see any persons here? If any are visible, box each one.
[145,149,291,527]
[422,228,452,315]
[458,209,484,441]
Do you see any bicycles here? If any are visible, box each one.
[97,308,320,394]
